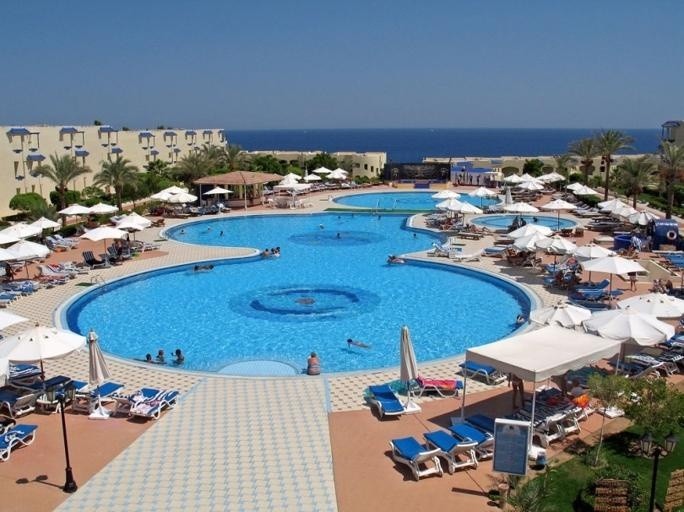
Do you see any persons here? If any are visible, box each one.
[413,233,417,238]
[320,223,325,230]
[387,255,399,264]
[306,351,320,375]
[261,244,281,259]
[139,347,185,366]
[336,232,342,238]
[507,373,527,407]
[345,337,372,350]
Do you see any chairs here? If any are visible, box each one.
[427,183,684,309]
[0,197,231,306]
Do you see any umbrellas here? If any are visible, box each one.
[0,320,86,380]
[398,324,420,397]
[87,327,108,411]
[0,185,234,280]
[277,166,350,198]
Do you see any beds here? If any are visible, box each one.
[2,375,33,414]
[130,391,178,418]
[116,385,158,418]
[53,380,84,401]
[74,382,124,412]
[1,424,36,460]
[22,375,69,408]
